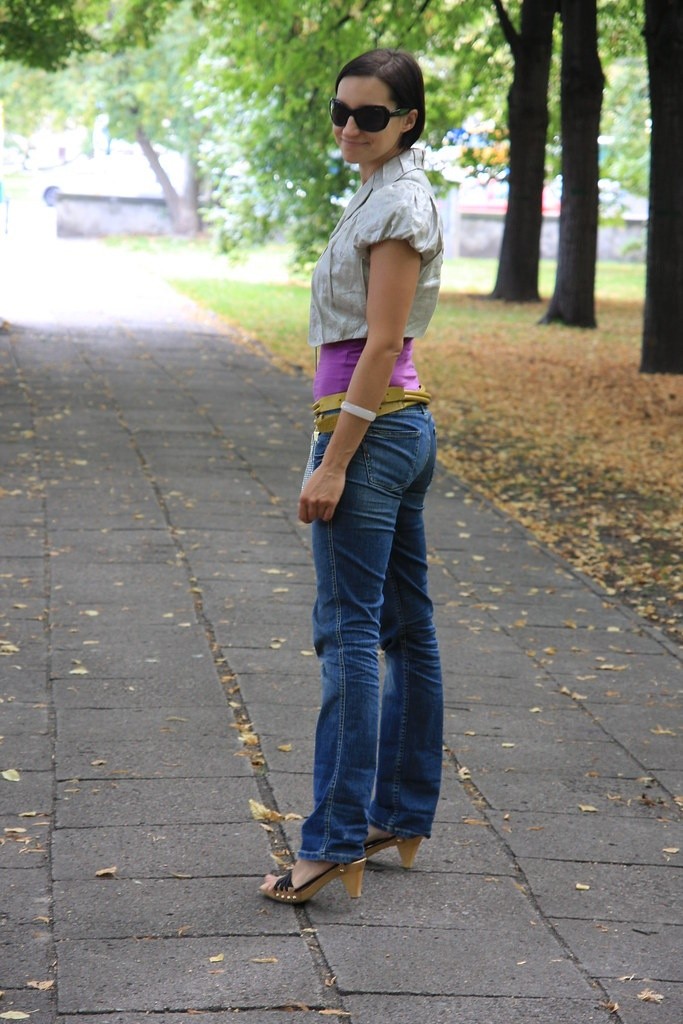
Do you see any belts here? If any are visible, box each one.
[312,385,433,432]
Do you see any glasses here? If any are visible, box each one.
[328,97,410,132]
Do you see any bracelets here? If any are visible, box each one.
[340,401,377,422]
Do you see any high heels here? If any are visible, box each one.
[364,834,424,869]
[259,857,367,904]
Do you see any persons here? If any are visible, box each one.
[257,49,445,901]
